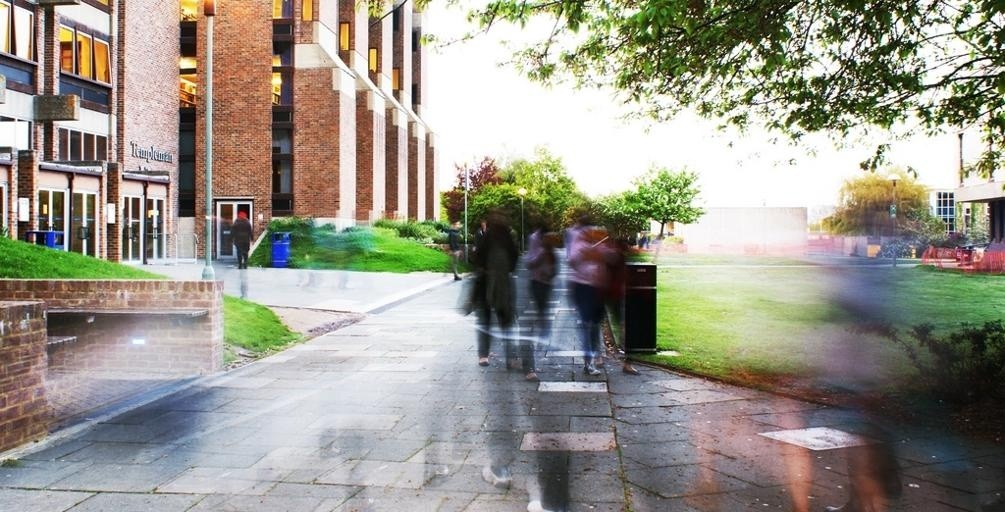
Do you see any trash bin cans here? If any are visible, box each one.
[25,230,64,248]
[270,231,291,267]
[622,262,657,354]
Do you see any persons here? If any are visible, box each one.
[231,211,254,268]
[448,212,662,379]
[954,245,959,260]
[986,237,1005,251]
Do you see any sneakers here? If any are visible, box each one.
[478,352,640,382]
[481,458,513,490]
[523,498,555,512]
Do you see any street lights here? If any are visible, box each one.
[518,187,527,253]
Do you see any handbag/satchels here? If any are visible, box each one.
[455,271,483,317]
